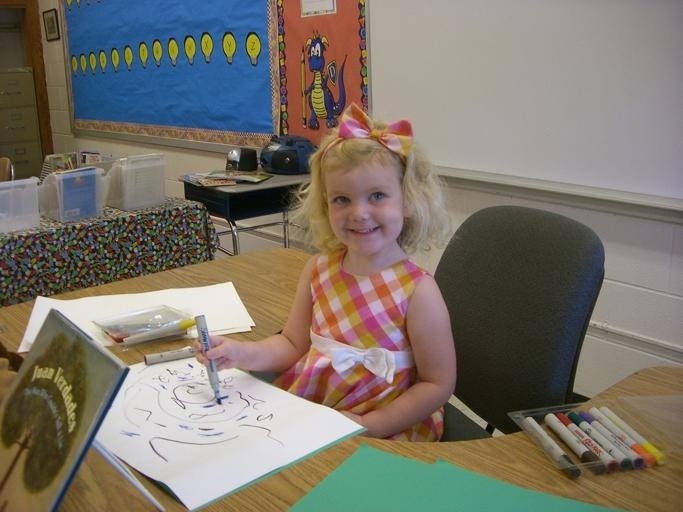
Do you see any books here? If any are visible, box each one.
[0,307,131,512]
[177,166,274,188]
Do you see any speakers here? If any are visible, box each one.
[226,148,258,172]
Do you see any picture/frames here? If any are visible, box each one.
[57,0,278,159]
[42,8,60,41]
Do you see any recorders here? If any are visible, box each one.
[260,135,319,174]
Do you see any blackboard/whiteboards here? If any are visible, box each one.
[372,2,683,214]
[59,2,370,162]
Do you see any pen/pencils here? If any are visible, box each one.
[122,318,198,365]
[521,406,667,480]
[194,315,221,404]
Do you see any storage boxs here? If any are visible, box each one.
[39,167,104,224]
[0,176,41,235]
[104,154,165,212]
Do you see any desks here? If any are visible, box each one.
[0,194,221,306]
[0,247,683,512]
[179,170,310,256]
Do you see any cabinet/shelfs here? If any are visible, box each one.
[1,67,44,180]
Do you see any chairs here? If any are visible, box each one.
[430,205,605,442]
[0,157,15,183]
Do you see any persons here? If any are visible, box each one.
[189,100,461,447]
